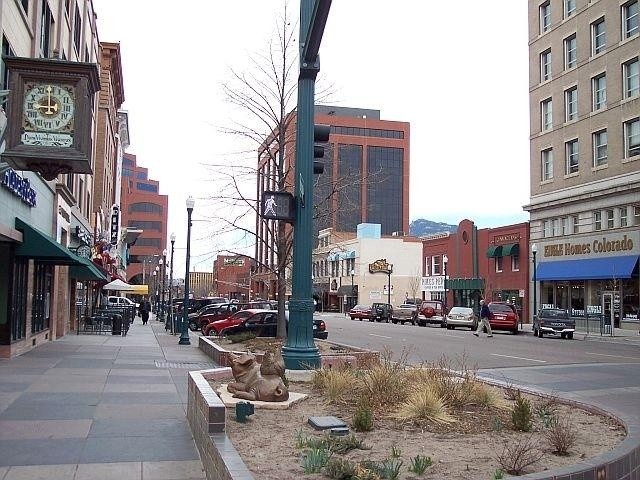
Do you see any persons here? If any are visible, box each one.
[473,300,493,338]
[101,295,109,305]
[140,298,151,325]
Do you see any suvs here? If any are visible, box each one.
[369,303,393,323]
[418,301,448,328]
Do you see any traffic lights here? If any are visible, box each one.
[261,190,295,221]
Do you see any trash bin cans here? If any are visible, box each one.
[112,314,122,335]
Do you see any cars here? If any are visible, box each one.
[350,304,372,321]
[534,308,575,339]
[174,297,328,340]
[107,296,140,310]
[447,307,479,331]
[484,301,520,335]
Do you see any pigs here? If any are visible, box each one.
[225,349,289,402]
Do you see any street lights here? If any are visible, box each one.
[531,244,538,324]
[153,232,176,327]
[351,269,355,308]
[387,265,392,309]
[444,256,448,315]
[178,195,195,341]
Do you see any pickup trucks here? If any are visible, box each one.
[392,304,419,325]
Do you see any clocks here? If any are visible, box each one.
[0,53,102,182]
